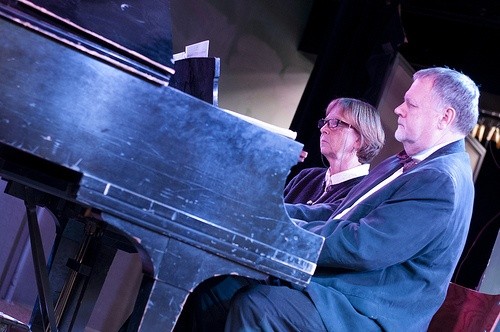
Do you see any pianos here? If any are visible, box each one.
[0,1,326,332]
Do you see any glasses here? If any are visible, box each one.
[317,118,360,134]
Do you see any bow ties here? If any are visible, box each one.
[399,156,415,172]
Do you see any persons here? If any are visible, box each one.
[175,63,479,331]
[280,97,385,208]
